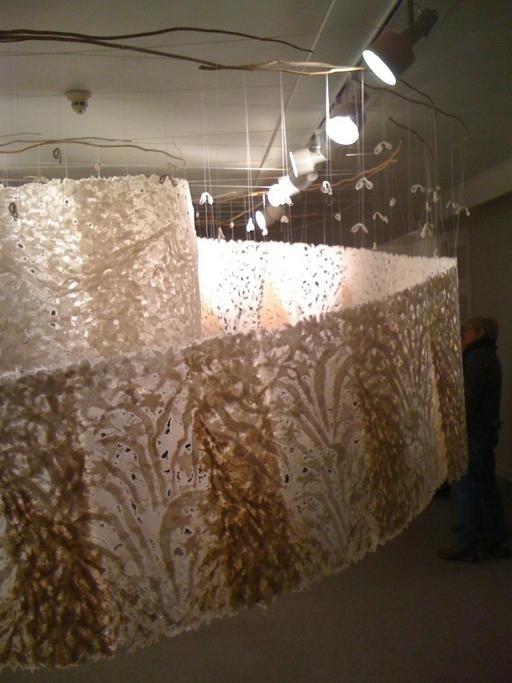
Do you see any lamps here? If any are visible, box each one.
[252,1,471,233]
[2,175,468,671]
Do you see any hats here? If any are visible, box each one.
[469,318,498,340]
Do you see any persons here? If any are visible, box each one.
[435,315,512,562]
